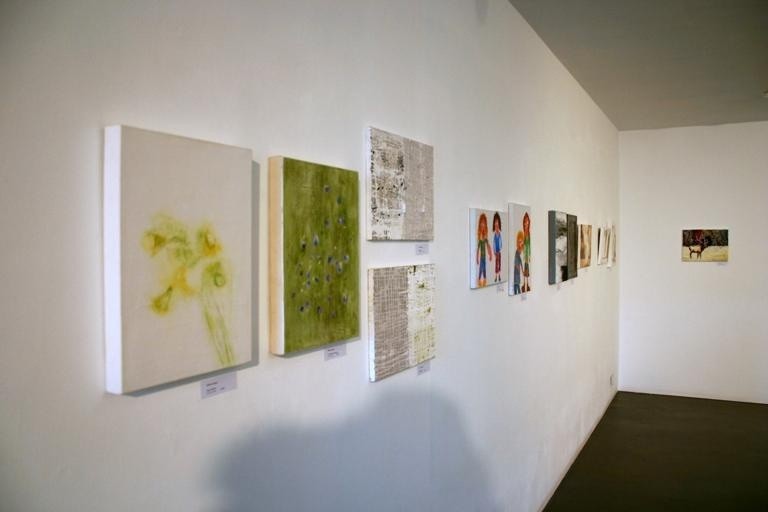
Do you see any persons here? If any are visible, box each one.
[474,211,531,295]
[556,226,568,282]
